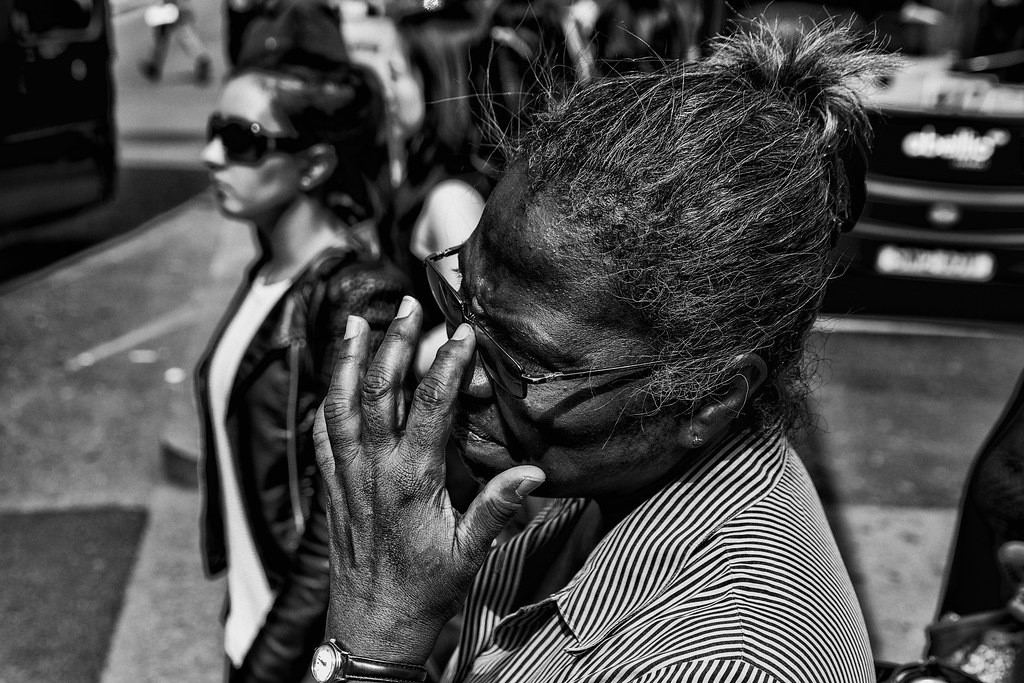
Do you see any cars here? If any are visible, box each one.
[819,57,1024,327]
[1,0,120,232]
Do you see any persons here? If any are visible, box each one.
[193,0,1024,683]
[134,0,213,88]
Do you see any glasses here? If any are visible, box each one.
[424,244,745,399]
[207,111,314,163]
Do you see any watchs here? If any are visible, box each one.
[310,637,428,683]
